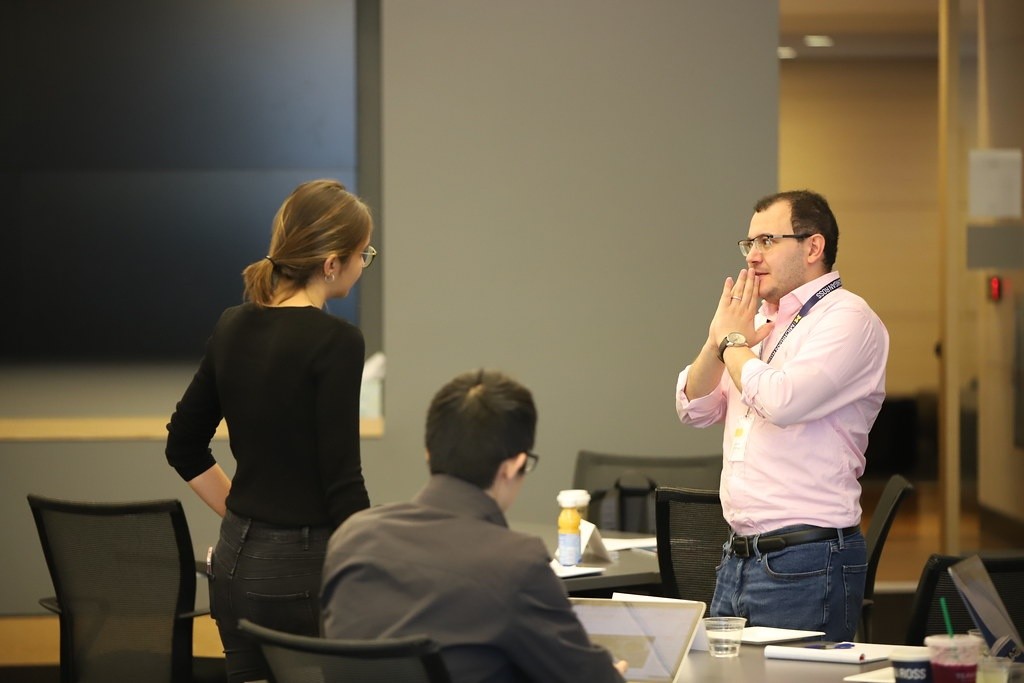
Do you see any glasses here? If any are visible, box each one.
[737,233,814,257]
[523,450,539,474]
[358,245,377,268]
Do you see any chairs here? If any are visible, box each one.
[27,493,210,683]
[904,552,1024,646]
[850,474,914,643]
[655,487,730,620]
[236,619,455,683]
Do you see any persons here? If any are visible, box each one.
[317,368,626,683]
[674,190,890,640]
[163,177,376,683]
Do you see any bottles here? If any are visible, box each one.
[557,502,581,565]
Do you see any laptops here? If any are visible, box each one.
[948,554,1024,664]
[568,597,707,683]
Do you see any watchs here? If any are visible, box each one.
[716,331,751,364]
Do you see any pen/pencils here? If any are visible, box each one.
[803,643,856,649]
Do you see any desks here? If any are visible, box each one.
[672,641,926,682]
[503,523,701,598]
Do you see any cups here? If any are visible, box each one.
[702,617,747,658]
[556,489,591,520]
[891,635,1010,683]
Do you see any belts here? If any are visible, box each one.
[725,525,864,558]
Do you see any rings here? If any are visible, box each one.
[731,295,741,301]
